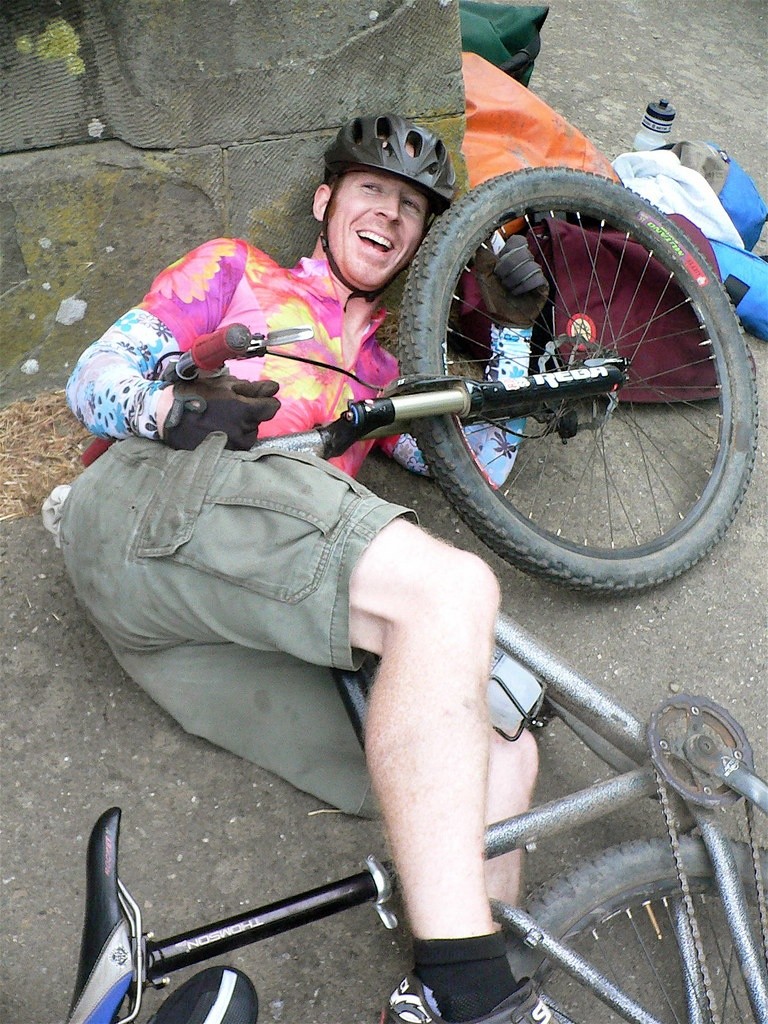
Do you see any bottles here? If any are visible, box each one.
[632,99,676,152]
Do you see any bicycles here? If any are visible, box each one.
[59,165,768,1024]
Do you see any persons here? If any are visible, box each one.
[62,114,558,1024]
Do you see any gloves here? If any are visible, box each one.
[475,234,549,328]
[162,375,281,451]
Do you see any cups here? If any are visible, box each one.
[489,645,542,733]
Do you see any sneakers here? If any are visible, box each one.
[385,974,560,1024]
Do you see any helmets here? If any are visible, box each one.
[323,113,456,204]
